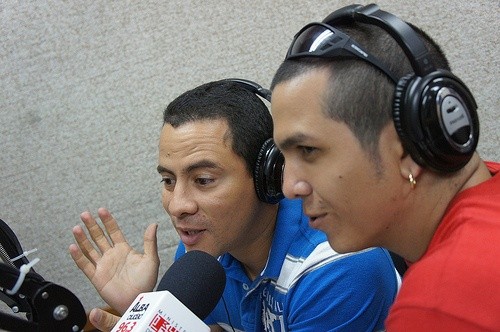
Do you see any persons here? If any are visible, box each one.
[268,4,500,331]
[70,78,403,332]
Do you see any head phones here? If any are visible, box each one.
[224,78,286,203]
[318,3,481,175]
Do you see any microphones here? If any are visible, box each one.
[109,251,226,332]
